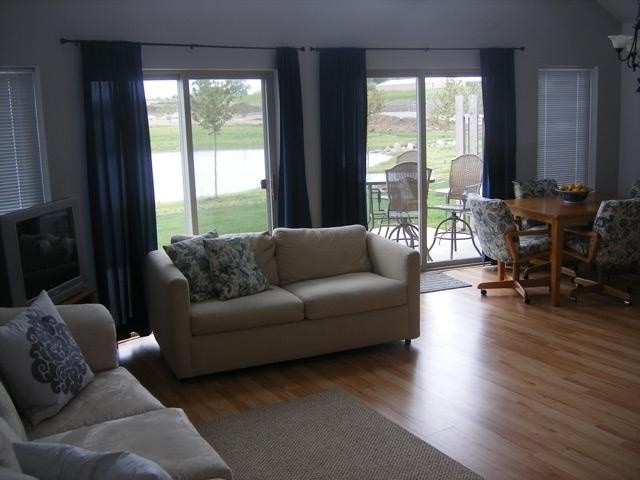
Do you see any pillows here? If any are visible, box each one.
[1,291,95,426]
[162,228,220,303]
[203,236,272,301]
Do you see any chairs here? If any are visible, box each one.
[429,154,484,259]
[396,149,418,164]
[467,192,551,302]
[512,178,578,280]
[627,180,639,198]
[564,198,640,307]
[385,161,433,263]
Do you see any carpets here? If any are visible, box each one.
[419,270,472,294]
[197,388,483,479]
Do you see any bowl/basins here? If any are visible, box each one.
[554,189,589,204]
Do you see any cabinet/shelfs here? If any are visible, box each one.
[61,286,98,304]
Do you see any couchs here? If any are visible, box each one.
[0,303,235,479]
[146,223,421,380]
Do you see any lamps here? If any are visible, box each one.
[608,11,640,91]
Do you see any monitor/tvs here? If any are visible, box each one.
[0,196,88,307]
